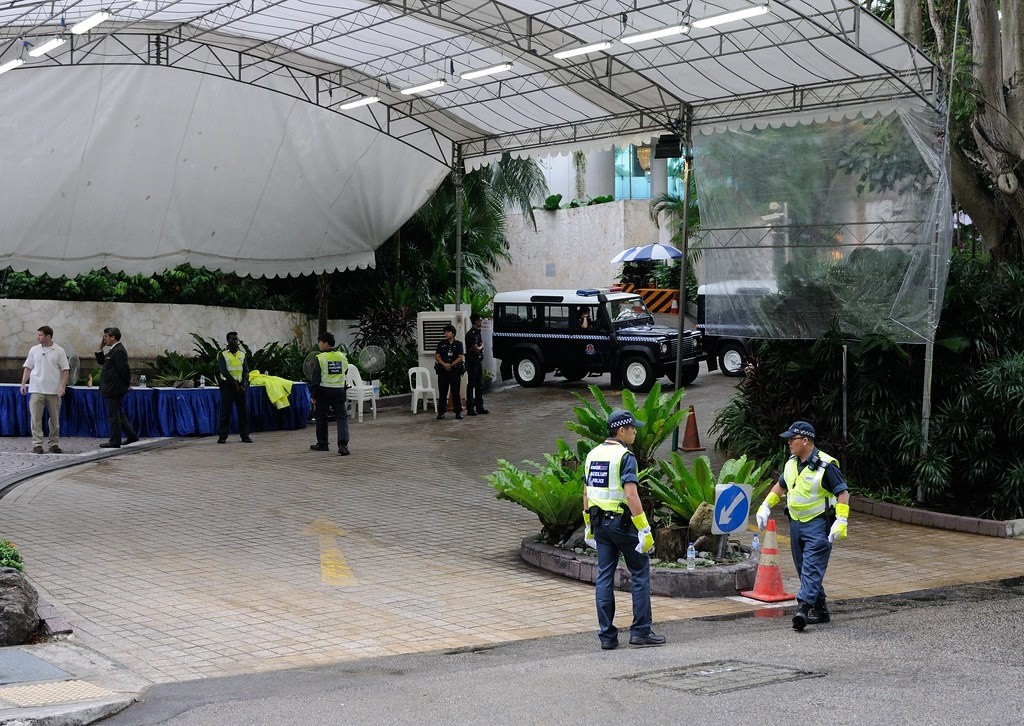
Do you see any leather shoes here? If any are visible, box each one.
[121,435,139,445]
[477,409,490,414]
[311,442,330,451]
[99,440,120,448]
[436,413,444,419]
[467,410,477,415]
[628,630,666,647]
[602,638,619,648]
[456,413,463,419]
[338,445,350,454]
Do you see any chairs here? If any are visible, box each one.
[345,364,377,422]
[595,309,603,329]
[408,367,438,414]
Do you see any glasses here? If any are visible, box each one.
[788,436,810,442]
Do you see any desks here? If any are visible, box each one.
[0,381,310,438]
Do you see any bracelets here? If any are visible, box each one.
[585,316,586,317]
[450,364,455,369]
[61,384,66,388]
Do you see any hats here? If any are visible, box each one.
[607,410,645,428]
[779,421,815,437]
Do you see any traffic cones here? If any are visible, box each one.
[742,519,799,603]
[679,405,706,451]
[669,293,679,315]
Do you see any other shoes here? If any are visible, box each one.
[217,438,225,443]
[49,445,62,453]
[242,435,253,443]
[34,446,44,453]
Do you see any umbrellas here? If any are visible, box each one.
[610,243,683,263]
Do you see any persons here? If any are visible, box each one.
[20,326,70,454]
[310,332,350,455]
[577,308,591,328]
[95,327,139,448]
[582,409,666,650]
[755,421,850,631]
[433,325,465,419]
[465,313,490,416]
[215,332,253,444]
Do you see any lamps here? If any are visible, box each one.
[28,37,67,58]
[553,39,614,59]
[654,134,683,159]
[399,78,447,95]
[339,96,381,110]
[0,57,26,75]
[70,9,113,35]
[620,22,691,45]
[691,3,770,28]
[460,62,513,80]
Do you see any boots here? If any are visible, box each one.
[807,598,831,624]
[792,601,811,629]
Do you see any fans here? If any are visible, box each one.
[303,351,322,424]
[67,354,82,386]
[359,345,386,413]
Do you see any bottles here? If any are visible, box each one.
[687,542,695,570]
[265,370,268,375]
[87,374,92,387]
[200,375,205,388]
[140,375,146,388]
[751,533,760,561]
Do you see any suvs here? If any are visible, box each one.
[695,279,783,378]
[492,286,708,393]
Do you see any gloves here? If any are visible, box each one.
[828,504,849,543]
[582,510,598,549]
[630,511,655,553]
[756,492,780,532]
